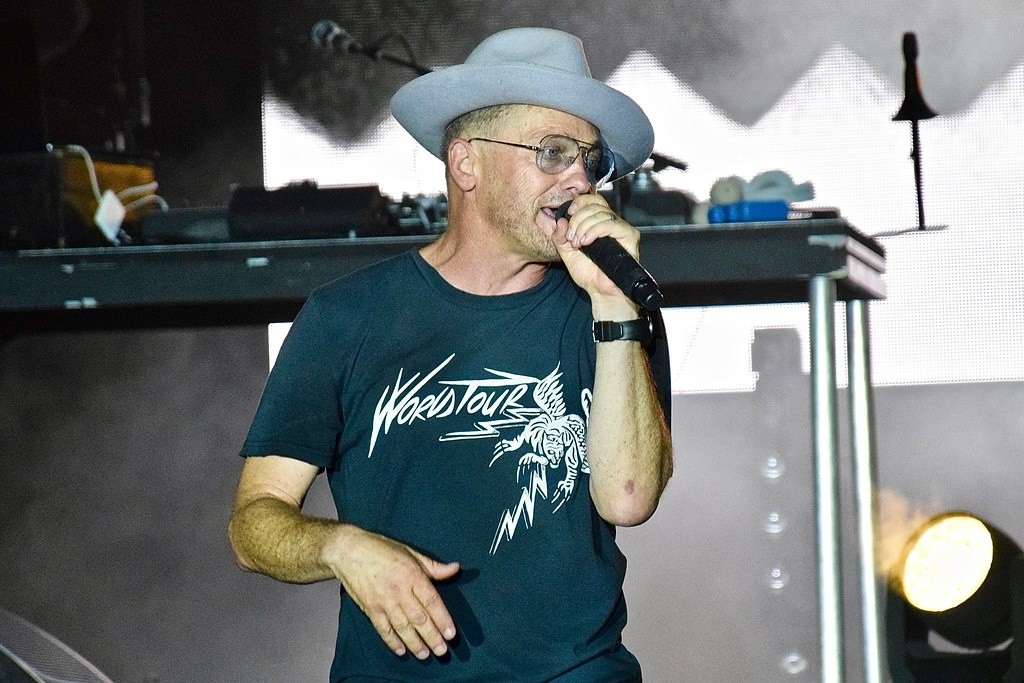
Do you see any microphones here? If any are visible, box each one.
[310,18,363,54]
[555,199,665,312]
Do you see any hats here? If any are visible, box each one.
[388,26,655,185]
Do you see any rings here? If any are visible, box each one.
[608,212,615,221]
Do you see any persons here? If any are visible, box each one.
[227,27,676,683]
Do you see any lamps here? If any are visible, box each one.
[885,512,1024,683]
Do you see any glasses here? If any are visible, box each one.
[467,134,617,189]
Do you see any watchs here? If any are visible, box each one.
[590,311,657,355]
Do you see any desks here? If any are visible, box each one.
[1,214,887,683]
